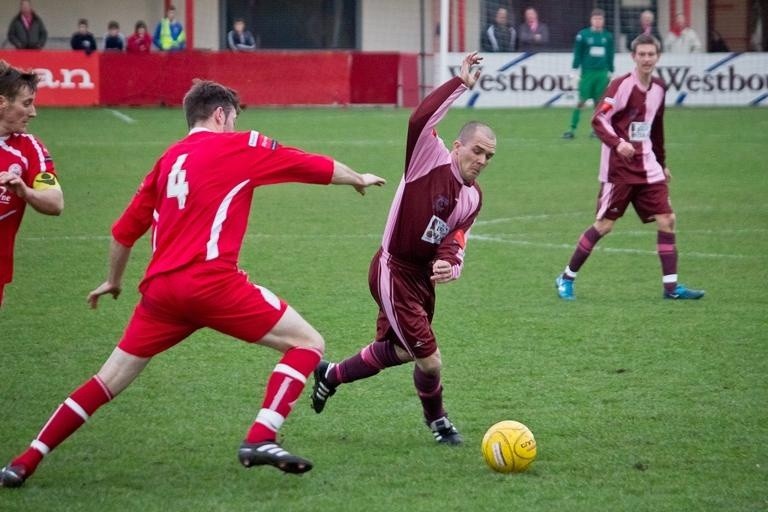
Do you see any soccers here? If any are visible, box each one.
[481,420,538,473]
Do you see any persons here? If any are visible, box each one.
[555,34,706,301]
[627,10,663,52]
[308,52,497,446]
[0,78,387,488]
[152,5,186,50]
[7,0,48,49]
[225,16,256,53]
[663,14,702,54]
[101,20,127,51]
[126,21,152,51]
[0,59,64,305]
[481,6,518,52]
[70,19,97,54]
[561,9,615,139]
[516,6,552,51]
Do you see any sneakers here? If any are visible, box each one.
[661,284,705,300]
[310,360,336,414]
[555,273,575,301]
[1,461,29,490]
[423,413,464,445]
[558,131,576,139]
[238,438,315,476]
